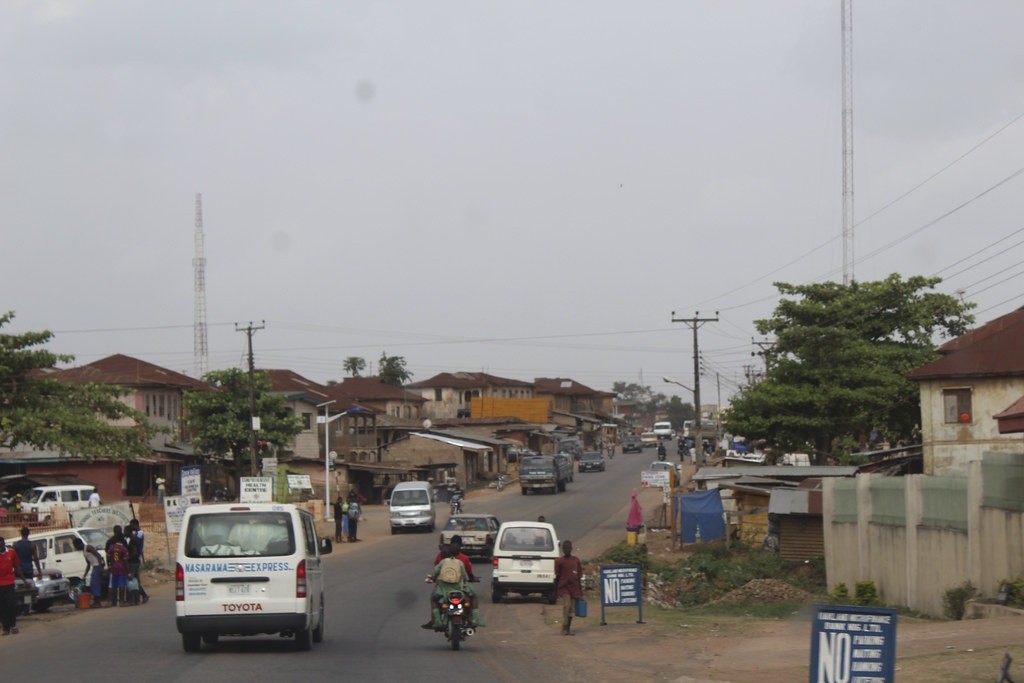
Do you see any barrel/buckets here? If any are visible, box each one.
[575,599,586,617]
[78,594,91,608]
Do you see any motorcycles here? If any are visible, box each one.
[496,474,504,491]
[450,502,461,514]
[608,446,614,458]
[434,576,486,650]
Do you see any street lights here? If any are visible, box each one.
[325,407,375,519]
[662,375,703,469]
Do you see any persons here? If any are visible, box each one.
[421,535,486,629]
[0,537,28,636]
[450,484,464,514]
[334,490,363,543]
[382,472,390,487]
[88,489,102,509]
[553,541,583,635]
[74,519,149,606]
[567,435,715,465]
[12,527,42,615]
[142,473,167,506]
[531,516,549,545]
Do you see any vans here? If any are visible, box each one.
[491,521,561,605]
[3,527,112,603]
[552,454,572,464]
[387,481,436,535]
[559,439,582,458]
[175,503,333,652]
[19,485,95,525]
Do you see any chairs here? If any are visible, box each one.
[507,535,516,546]
[55,544,61,554]
[63,543,72,553]
[533,536,545,546]
[395,493,406,504]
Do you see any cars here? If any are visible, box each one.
[621,421,691,462]
[649,462,681,487]
[12,577,38,608]
[578,451,605,472]
[31,569,70,612]
[438,514,500,557]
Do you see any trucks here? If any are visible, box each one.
[515,456,572,495]
